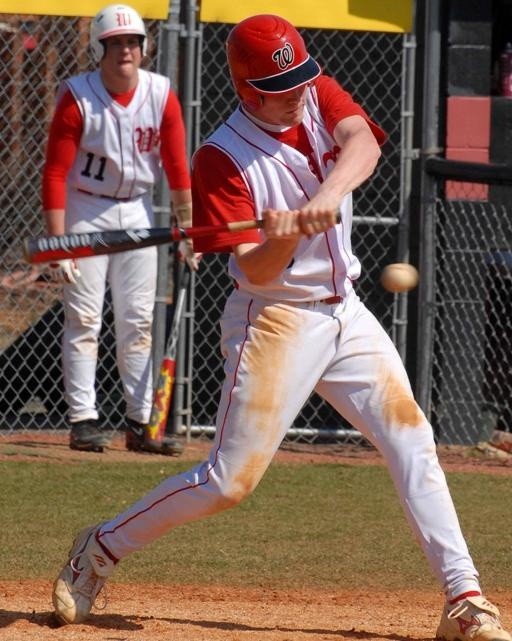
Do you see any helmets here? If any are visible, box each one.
[90,4,148,63]
[225,14,322,111]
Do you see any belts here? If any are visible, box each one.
[77,188,141,202]
[233,279,342,307]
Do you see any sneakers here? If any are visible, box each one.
[125,421,183,456]
[435,595,512,641]
[52,521,120,626]
[69,419,111,449]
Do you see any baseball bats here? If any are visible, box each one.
[144,263,189,446]
[22,210,343,263]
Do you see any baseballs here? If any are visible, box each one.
[383,264,417,293]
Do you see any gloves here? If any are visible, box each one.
[177,238,204,272]
[48,258,82,285]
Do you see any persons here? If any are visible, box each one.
[52,15,512,641]
[42,6,202,454]
